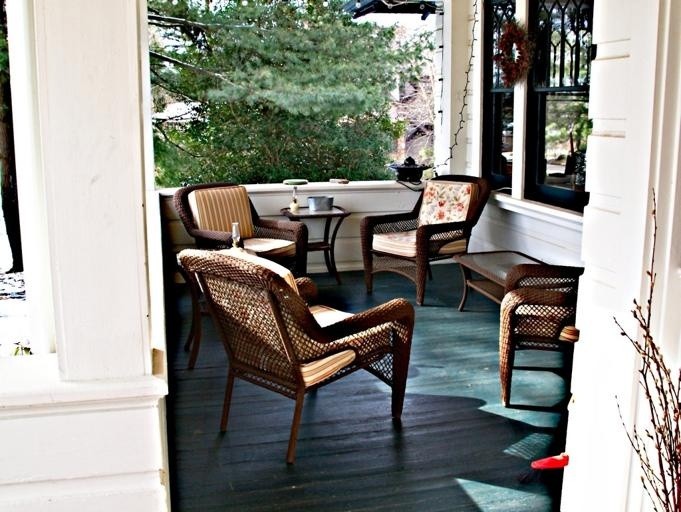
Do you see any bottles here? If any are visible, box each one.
[290,186,300,213]
[232,223,241,247]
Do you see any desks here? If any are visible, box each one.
[453,250,549,312]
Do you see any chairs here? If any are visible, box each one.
[172,183,308,279]
[498,264,586,408]
[360,175,491,305]
[177,248,415,463]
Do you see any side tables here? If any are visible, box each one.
[280,204,351,286]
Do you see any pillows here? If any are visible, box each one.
[211,248,301,296]
[418,179,480,233]
[187,185,254,238]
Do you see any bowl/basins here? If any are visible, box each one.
[307,197,334,211]
[390,165,432,182]
